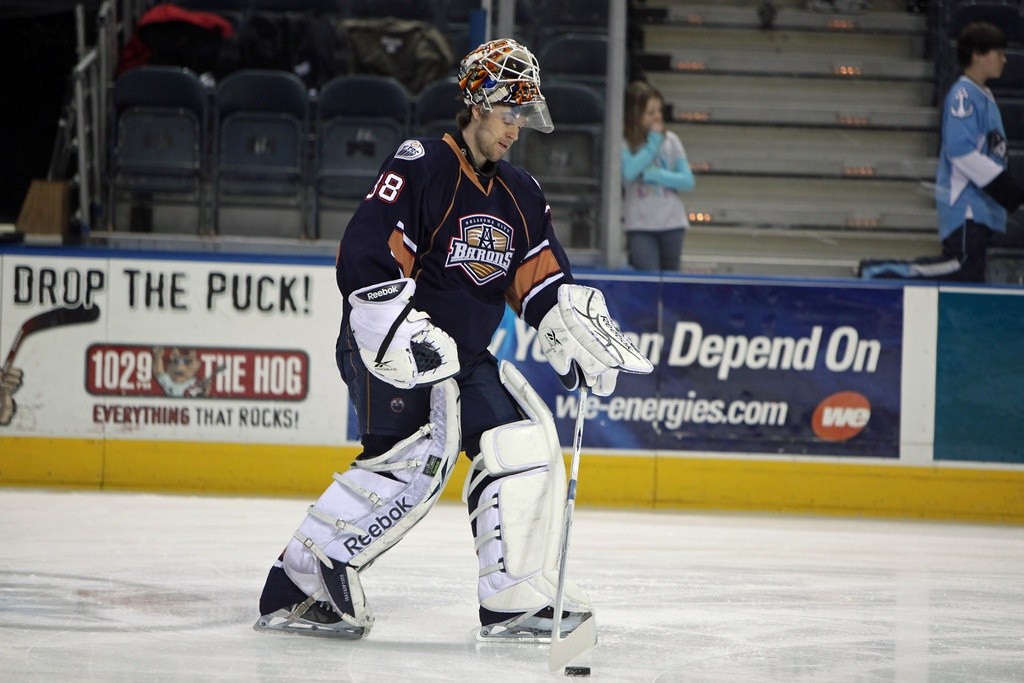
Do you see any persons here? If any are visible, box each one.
[622,81,694,271]
[857,22,1024,282]
[254,38,654,646]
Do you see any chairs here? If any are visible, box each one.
[111,0,612,248]
[939,0,1024,234]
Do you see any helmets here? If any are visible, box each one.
[454,37,554,134]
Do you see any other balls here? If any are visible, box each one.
[564,665,592,677]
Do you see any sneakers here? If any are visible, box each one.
[252,587,371,639]
[478,603,591,640]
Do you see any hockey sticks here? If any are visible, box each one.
[543,380,601,674]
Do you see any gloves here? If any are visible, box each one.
[536,281,653,397]
[349,278,460,391]
